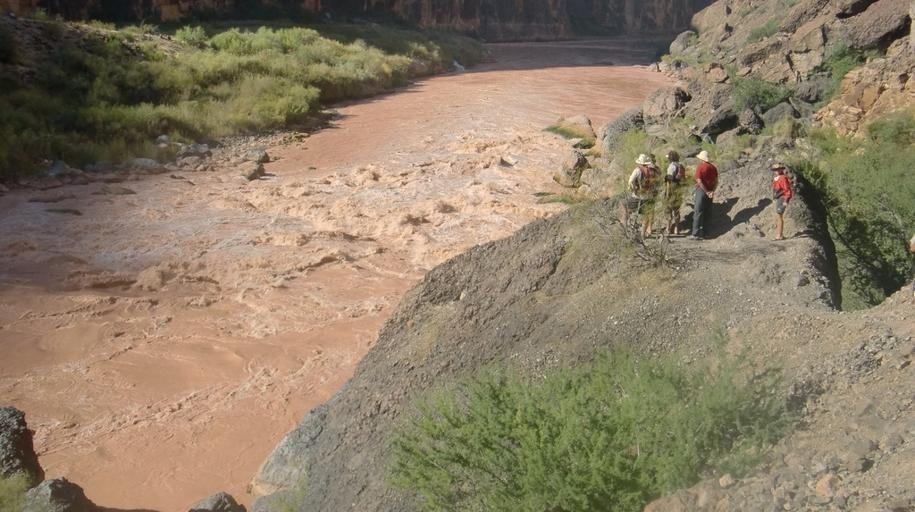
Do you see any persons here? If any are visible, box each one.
[648,153,661,237]
[661,151,686,234]
[687,150,719,239]
[770,163,791,240]
[621,154,653,240]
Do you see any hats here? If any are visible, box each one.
[771,164,786,170]
[696,151,709,163]
[635,154,652,165]
